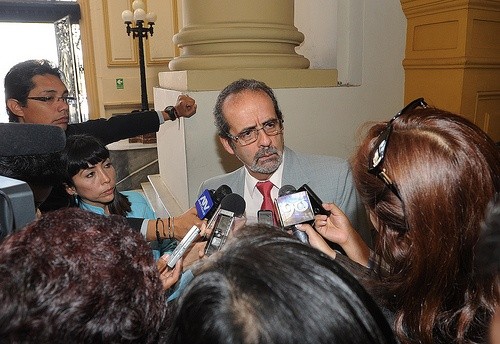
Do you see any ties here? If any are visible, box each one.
[256,181,280,227]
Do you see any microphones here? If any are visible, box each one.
[204,192,245,258]
[0,123,67,157]
[204,184,233,220]
[274,185,315,245]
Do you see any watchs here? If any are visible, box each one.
[164,106,176,121]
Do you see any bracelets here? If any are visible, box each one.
[175,109,179,118]
[156,217,175,243]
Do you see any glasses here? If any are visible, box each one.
[368,97,432,205]
[226,119,284,146]
[26,93,77,105]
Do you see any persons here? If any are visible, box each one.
[54,134,210,241]
[173,224,398,344]
[287,98,500,344]
[0,208,183,344]
[314,203,391,274]
[4,59,196,146]
[197,79,376,255]
